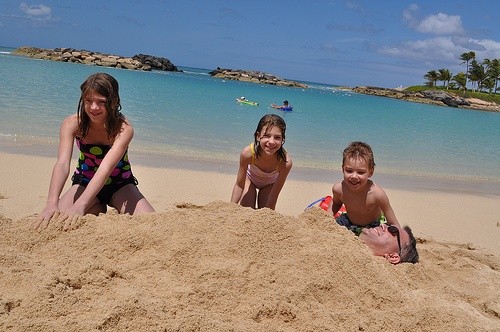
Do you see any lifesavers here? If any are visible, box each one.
[277,106,293,111]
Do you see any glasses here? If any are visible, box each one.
[384,221,401,257]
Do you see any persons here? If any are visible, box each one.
[235,96,259,106]
[270,100,289,109]
[30,72,155,232]
[326,141,401,237]
[359,223,420,264]
[230,113,293,211]
